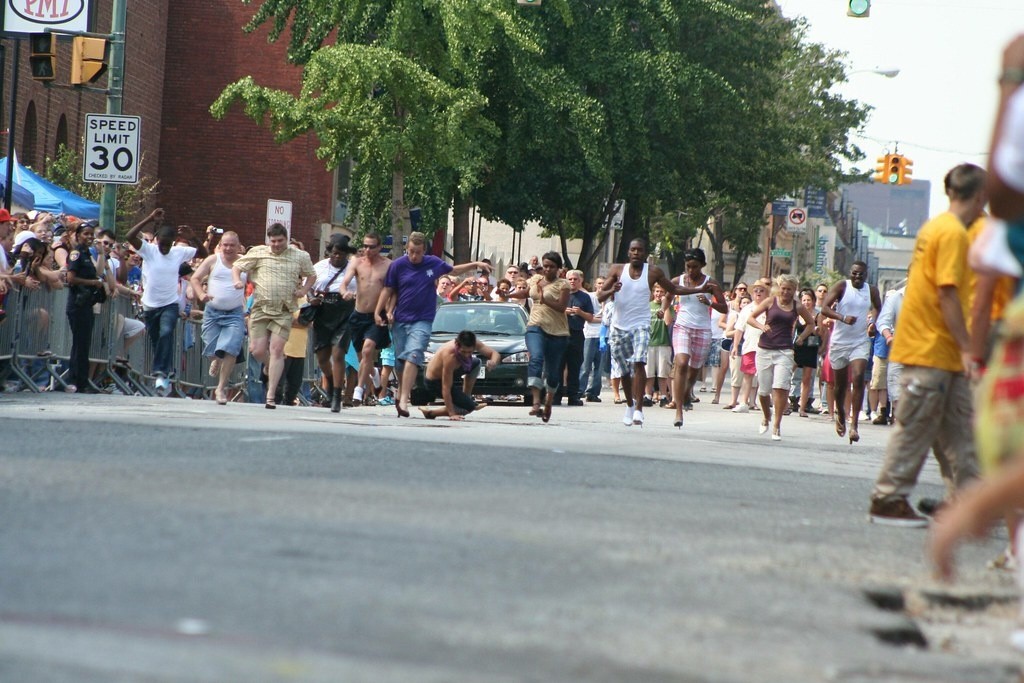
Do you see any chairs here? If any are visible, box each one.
[442,313,466,332]
[493,314,520,333]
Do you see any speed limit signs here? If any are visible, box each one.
[84,114,140,186]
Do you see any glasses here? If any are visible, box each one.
[457,266,530,296]
[81,223,95,227]
[364,243,378,249]
[734,287,748,292]
[851,271,866,276]
[104,241,113,247]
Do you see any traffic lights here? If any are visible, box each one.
[875,153,913,186]
[847,0,871,17]
[71,36,113,85]
[29,32,57,80]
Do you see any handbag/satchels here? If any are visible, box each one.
[93,286,107,303]
[297,303,317,325]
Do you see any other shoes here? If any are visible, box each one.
[5,355,198,402]
[918,498,946,516]
[265,398,276,408]
[314,372,476,420]
[209,360,219,377]
[526,381,894,443]
[215,389,227,403]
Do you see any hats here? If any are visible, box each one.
[0,209,18,221]
[68,217,75,222]
[15,231,37,245]
[11,212,28,220]
[27,211,37,219]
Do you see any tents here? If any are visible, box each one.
[0,155,101,219]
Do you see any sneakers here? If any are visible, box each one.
[869,498,929,527]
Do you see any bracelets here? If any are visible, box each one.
[868,320,876,325]
[841,315,846,321]
[998,67,1023,84]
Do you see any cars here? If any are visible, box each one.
[412,302,533,406]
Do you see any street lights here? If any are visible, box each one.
[791,68,900,275]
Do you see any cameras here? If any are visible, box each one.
[212,227,223,233]
[469,280,477,284]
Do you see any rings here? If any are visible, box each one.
[700,298,702,301]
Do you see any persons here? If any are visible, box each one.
[867,162,1015,527]
[0,208,317,409]
[436,250,595,423]
[303,232,498,418]
[577,237,729,429]
[723,261,882,445]
[922,32,1024,587]
[418,330,501,420]
